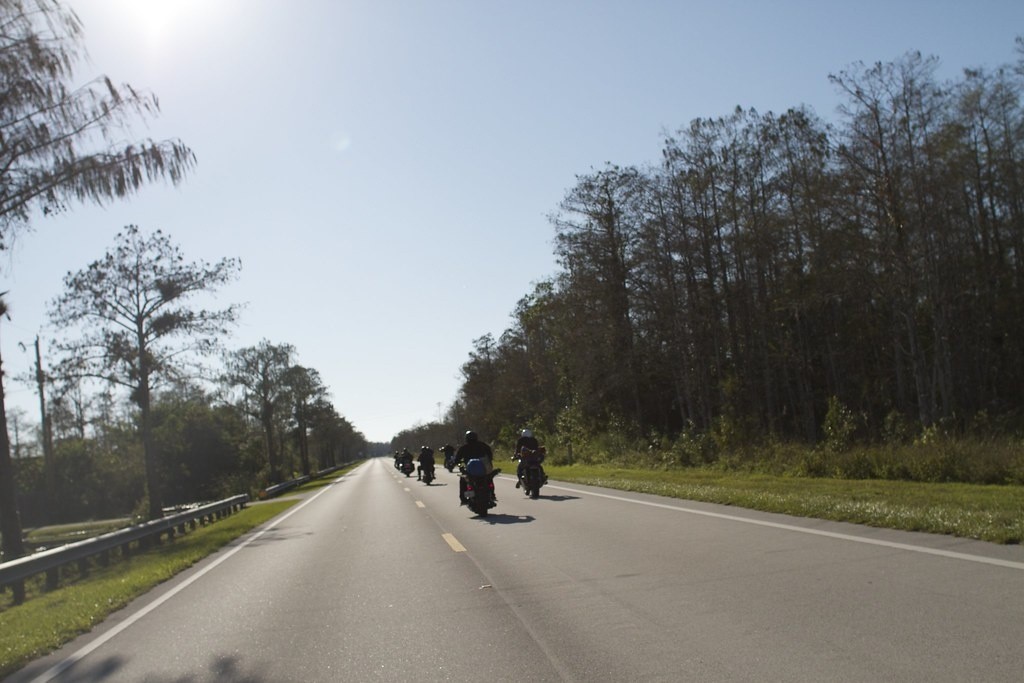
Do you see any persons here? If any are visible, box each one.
[394,448,414,473]
[417,446,436,481]
[513,429,548,489]
[447,430,493,505]
[438,444,456,468]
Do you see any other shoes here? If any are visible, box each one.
[417,478,421,481]
[516,481,521,488]
[433,477,436,479]
[461,498,467,505]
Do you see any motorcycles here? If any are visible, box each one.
[394,456,415,479]
[416,458,437,485]
[510,451,549,501]
[439,447,456,473]
[452,461,501,517]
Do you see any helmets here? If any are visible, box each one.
[404,447,407,451]
[521,428,533,438]
[421,445,430,451]
[396,450,399,454]
[445,444,449,447]
[465,431,477,443]
